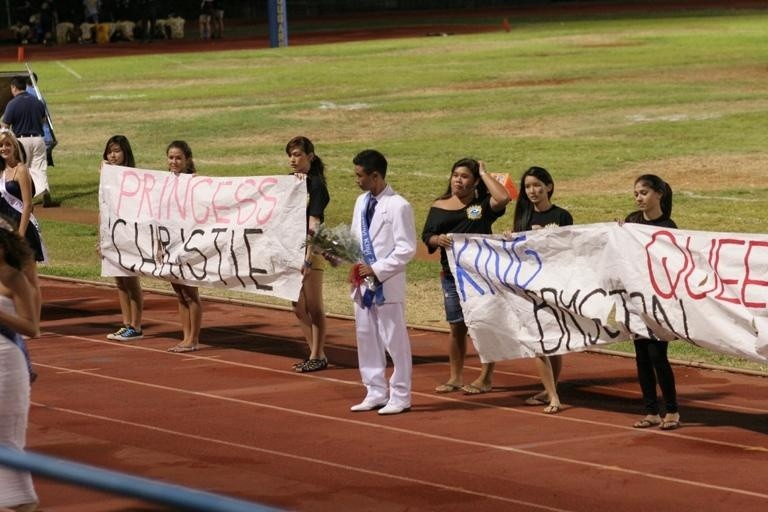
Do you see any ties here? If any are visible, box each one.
[365,198,376,227]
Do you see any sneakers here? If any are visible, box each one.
[167,344,185,352]
[296,359,328,372]
[377,397,411,415]
[30,194,42,204]
[41,191,52,207]
[107,328,128,340]
[350,399,384,412]
[174,345,200,352]
[115,328,144,341]
[289,359,309,371]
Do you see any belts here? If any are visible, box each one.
[16,132,43,138]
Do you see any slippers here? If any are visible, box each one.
[659,419,679,430]
[433,382,465,394]
[543,401,559,414]
[633,417,660,428]
[524,394,548,406]
[459,383,491,395]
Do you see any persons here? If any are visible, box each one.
[211,0,224,40]
[503,166,573,414]
[614,174,681,431]
[199,0,211,41]
[286,136,330,373]
[421,158,511,394]
[0,128,42,340]
[83,0,101,25]
[0,77,51,209]
[167,140,202,353]
[98,135,143,342]
[348,148,417,415]
[140,6,156,45]
[1,226,39,512]
